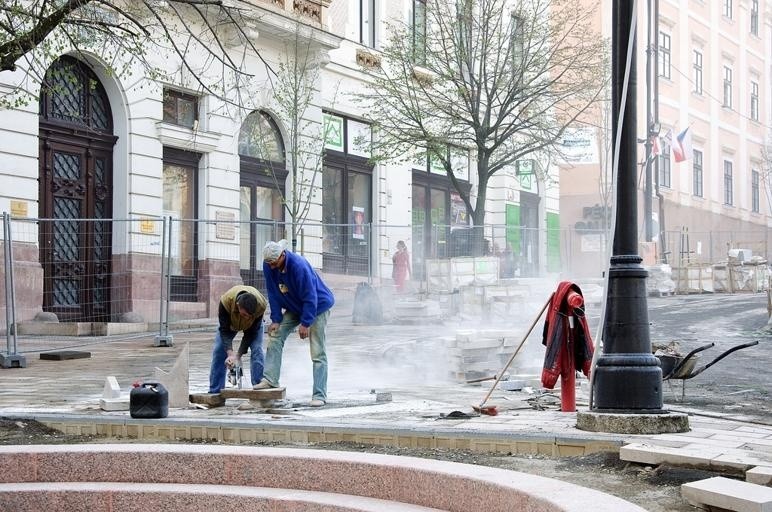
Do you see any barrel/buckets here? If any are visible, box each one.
[129,381,169,419]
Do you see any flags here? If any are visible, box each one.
[663,129,685,163]
[650,132,662,155]
[677,126,689,142]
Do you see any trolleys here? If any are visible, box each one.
[652,341,758,402]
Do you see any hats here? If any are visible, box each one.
[262,238,289,264]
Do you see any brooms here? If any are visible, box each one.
[471,292,555,417]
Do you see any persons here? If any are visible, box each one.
[209,283,268,390]
[392,240,410,292]
[481,238,518,278]
[253,239,335,406]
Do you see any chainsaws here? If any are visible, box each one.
[228,361,242,390]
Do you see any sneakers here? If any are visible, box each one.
[309,399,326,408]
[253,381,270,391]
